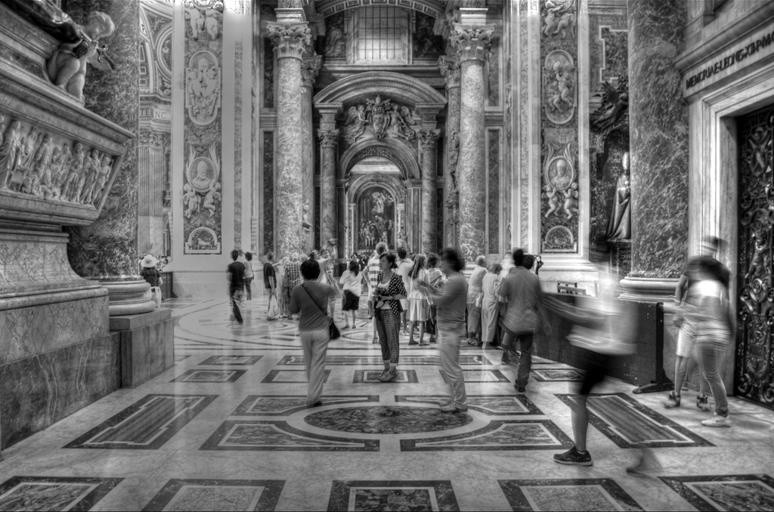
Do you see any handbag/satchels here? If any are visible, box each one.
[328,316,341,341]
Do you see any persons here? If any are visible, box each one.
[344,96,415,148]
[544,227,665,476]
[140,254,164,308]
[185,0,224,41]
[467,249,544,392]
[414,248,468,412]
[395,248,445,345]
[541,158,579,221]
[241,252,255,301]
[290,259,341,406]
[277,246,336,320]
[0,114,113,204]
[363,242,407,381]
[183,161,221,221]
[263,253,278,322]
[359,190,394,247]
[675,256,733,428]
[185,65,218,115]
[541,59,575,116]
[339,252,370,328]
[48,9,115,100]
[224,249,247,325]
[540,0,578,41]
[661,236,728,412]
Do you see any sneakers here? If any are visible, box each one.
[340,323,356,330]
[695,392,713,413]
[305,400,323,409]
[408,340,430,346]
[513,381,527,393]
[267,312,300,322]
[552,445,595,467]
[466,337,497,350]
[439,397,469,413]
[662,391,681,409]
[701,413,732,428]
[376,366,399,383]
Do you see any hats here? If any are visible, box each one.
[140,254,158,268]
